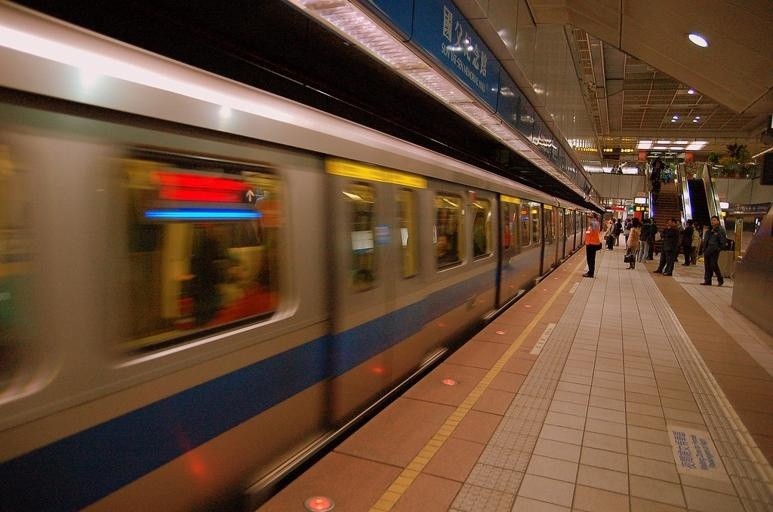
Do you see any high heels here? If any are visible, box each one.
[626,266,634,269]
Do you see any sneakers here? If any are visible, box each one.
[583,273,592,278]
[700,281,723,286]
[653,271,672,276]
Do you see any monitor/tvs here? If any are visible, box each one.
[634,197,647,204]
[720,201,730,210]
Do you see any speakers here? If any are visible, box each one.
[760,153,773,186]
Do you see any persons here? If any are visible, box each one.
[581,213,726,287]
[611,165,623,175]
[191,223,239,326]
[434,206,459,265]
[650,157,672,205]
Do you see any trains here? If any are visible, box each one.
[0,1,607,512]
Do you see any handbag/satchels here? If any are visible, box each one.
[596,241,602,251]
[623,229,630,236]
[604,236,609,240]
[655,232,661,242]
[624,251,635,262]
